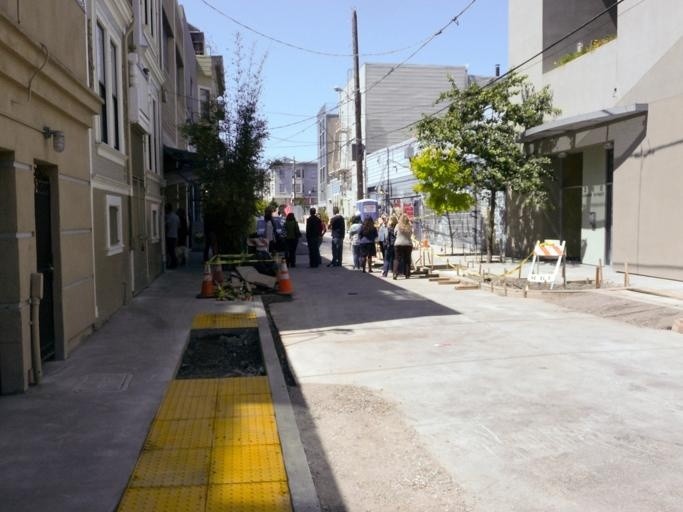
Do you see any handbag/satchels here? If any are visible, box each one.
[279,230,287,238]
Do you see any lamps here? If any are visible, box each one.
[46,125,64,155]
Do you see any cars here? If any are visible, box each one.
[256,215,286,242]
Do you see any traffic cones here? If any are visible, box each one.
[201,253,224,298]
[277,258,295,294]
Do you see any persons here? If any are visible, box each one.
[348,211,414,280]
[306,208,322,268]
[316,212,326,243]
[165,202,181,267]
[264,204,302,268]
[325,206,345,267]
[176,207,192,267]
